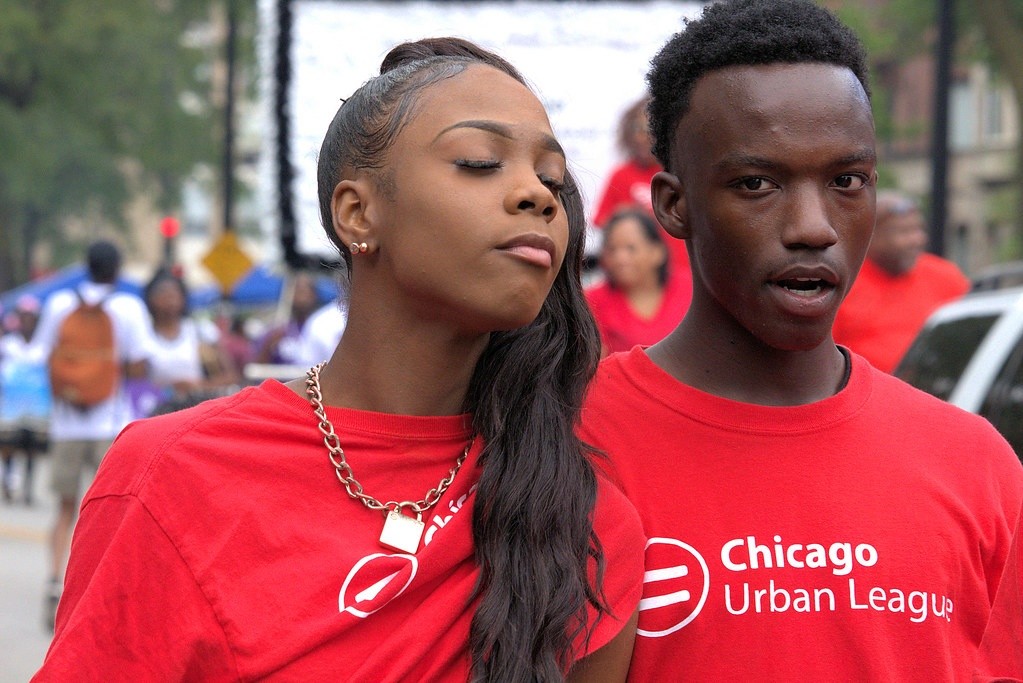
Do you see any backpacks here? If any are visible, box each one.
[49,286,120,411]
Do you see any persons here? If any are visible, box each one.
[0,0,1023,683]
